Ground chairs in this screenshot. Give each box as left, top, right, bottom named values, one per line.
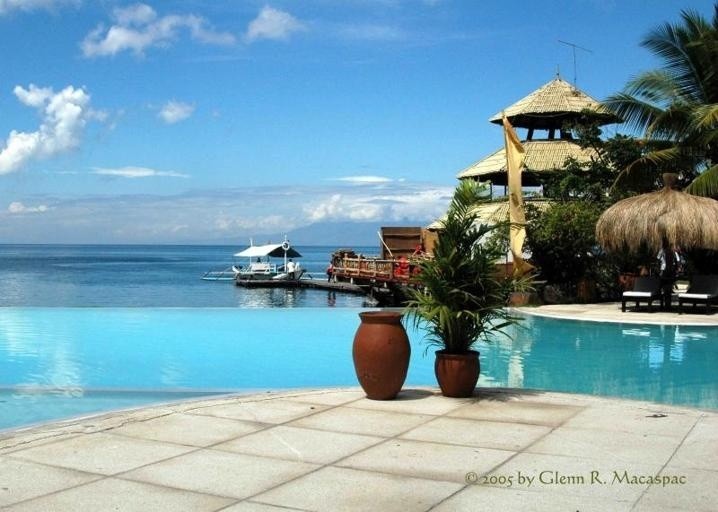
left=622, top=273, right=718, bottom=315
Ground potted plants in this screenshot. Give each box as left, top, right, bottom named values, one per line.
left=397, top=176, right=549, bottom=398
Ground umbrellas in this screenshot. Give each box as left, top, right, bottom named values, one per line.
left=596, top=184, right=718, bottom=312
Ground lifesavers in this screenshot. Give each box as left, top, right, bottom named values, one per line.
left=282, top=241, right=290, bottom=251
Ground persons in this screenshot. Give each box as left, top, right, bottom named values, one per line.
left=286, top=259, right=295, bottom=280
left=327, top=261, right=336, bottom=283
left=656, top=247, right=680, bottom=292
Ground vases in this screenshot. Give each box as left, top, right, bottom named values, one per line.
left=353, top=312, right=411, bottom=399
